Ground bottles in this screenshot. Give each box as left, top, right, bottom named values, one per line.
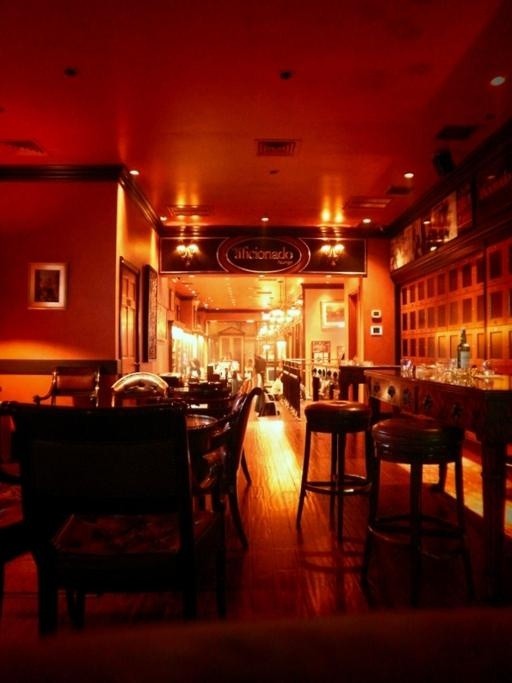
left=456, top=329, right=470, bottom=368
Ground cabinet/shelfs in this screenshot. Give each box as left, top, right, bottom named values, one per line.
left=280, top=358, right=305, bottom=415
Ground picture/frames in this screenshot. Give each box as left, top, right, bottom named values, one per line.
left=156, top=301, right=169, bottom=344
left=319, top=299, right=345, bottom=331
left=142, top=264, right=157, bottom=362
left=27, top=256, right=71, bottom=314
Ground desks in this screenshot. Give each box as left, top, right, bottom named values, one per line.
left=0, top=388, right=223, bottom=576
left=310, top=361, right=401, bottom=435
left=364, top=365, right=512, bottom=604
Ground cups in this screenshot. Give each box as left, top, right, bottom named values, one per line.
left=400, top=358, right=496, bottom=387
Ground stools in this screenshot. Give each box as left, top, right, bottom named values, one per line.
left=294, top=391, right=379, bottom=546
left=355, top=415, right=478, bottom=606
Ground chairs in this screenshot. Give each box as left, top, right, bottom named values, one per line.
left=11, top=388, right=217, bottom=637
left=32, top=364, right=99, bottom=406
left=191, top=370, right=268, bottom=558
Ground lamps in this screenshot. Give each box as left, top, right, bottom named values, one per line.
left=322, top=237, right=345, bottom=267
left=176, top=240, right=205, bottom=267
